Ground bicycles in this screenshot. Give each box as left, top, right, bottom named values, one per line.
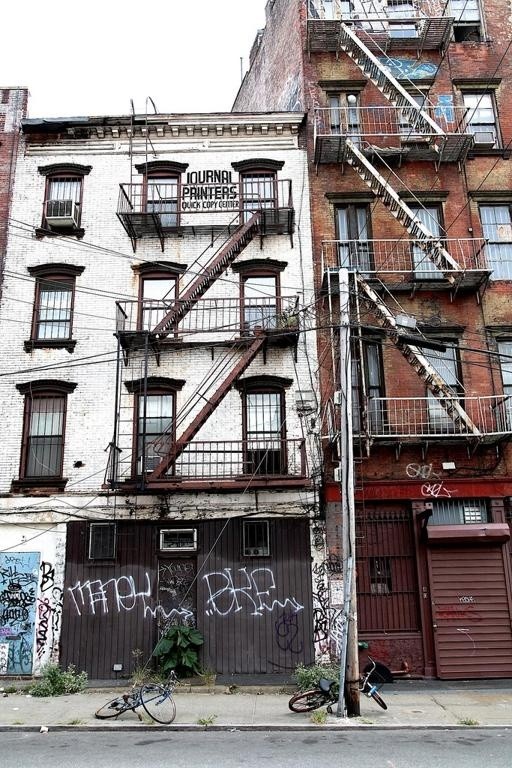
left=286, top=653, right=396, bottom=715
left=92, top=667, right=183, bottom=725
